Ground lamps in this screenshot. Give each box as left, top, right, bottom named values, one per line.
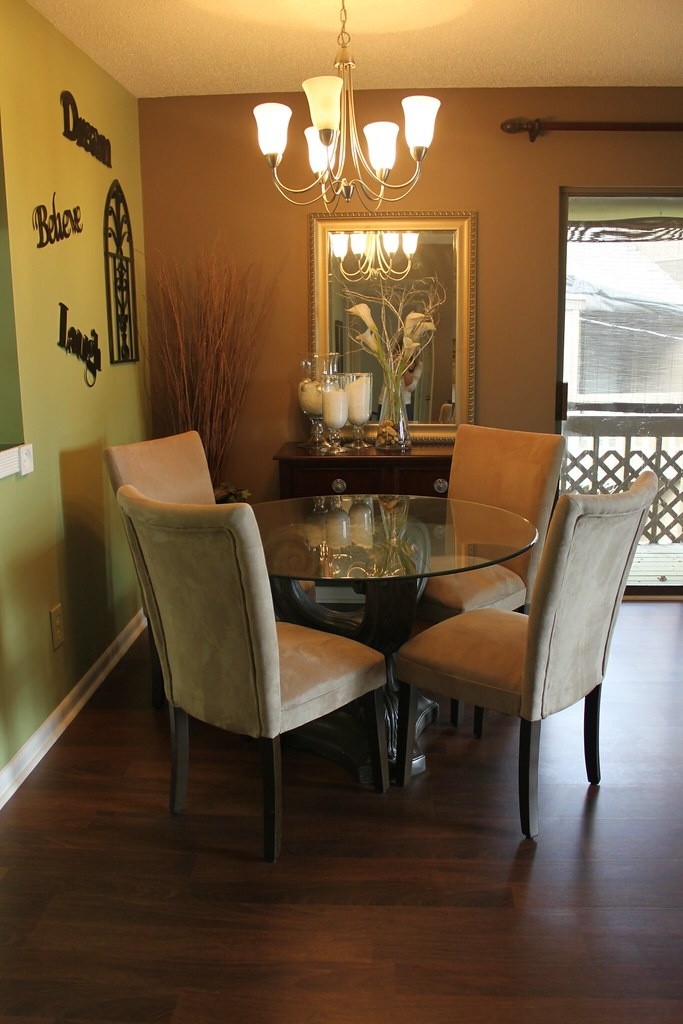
left=330, top=221, right=420, bottom=284
left=251, top=1, right=441, bottom=214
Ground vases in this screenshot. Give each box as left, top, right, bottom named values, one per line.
left=381, top=377, right=410, bottom=452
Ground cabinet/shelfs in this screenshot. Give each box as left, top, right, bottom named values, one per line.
left=272, top=441, right=452, bottom=499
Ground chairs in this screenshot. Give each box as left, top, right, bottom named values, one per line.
left=105, top=430, right=216, bottom=713
left=432, top=426, right=566, bottom=741
left=114, top=483, right=389, bottom=865
left=396, top=472, right=659, bottom=837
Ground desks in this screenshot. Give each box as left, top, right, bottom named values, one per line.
left=227, top=497, right=539, bottom=787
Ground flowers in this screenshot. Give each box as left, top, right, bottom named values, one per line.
left=346, top=289, right=442, bottom=373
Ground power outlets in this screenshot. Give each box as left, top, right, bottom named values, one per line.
left=51, top=603, right=66, bottom=650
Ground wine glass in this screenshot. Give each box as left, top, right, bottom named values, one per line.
left=306, top=495, right=374, bottom=552
left=321, top=374, right=349, bottom=454
left=294, top=351, right=340, bottom=449
left=343, top=371, right=373, bottom=450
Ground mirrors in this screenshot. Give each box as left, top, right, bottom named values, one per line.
left=310, top=214, right=478, bottom=447
